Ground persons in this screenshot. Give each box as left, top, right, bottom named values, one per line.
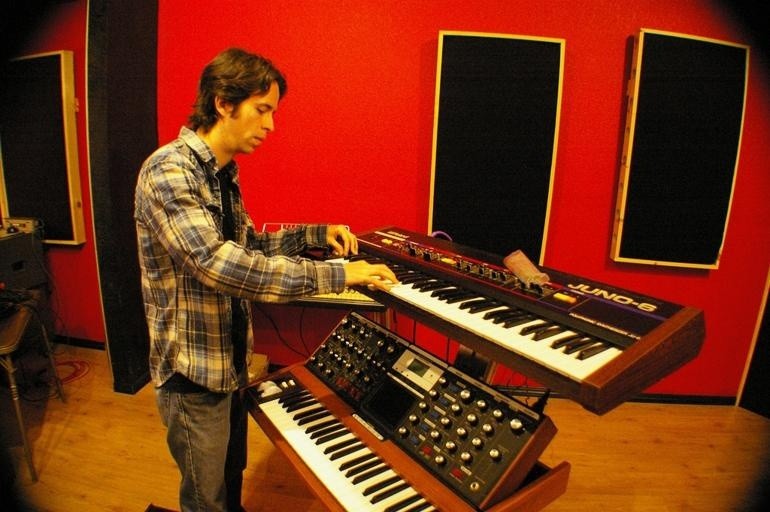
left=134, top=46, right=402, bottom=511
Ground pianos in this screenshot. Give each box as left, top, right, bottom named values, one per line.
left=237, top=227, right=706, bottom=512
